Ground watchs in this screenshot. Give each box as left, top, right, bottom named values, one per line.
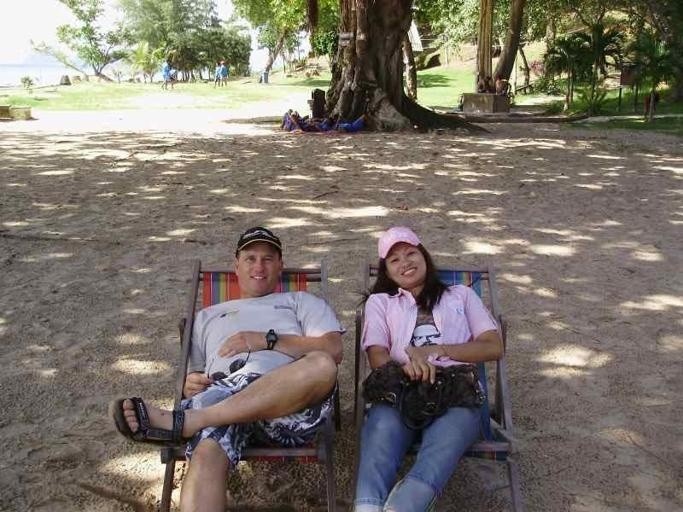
left=265, top=329, right=278, bottom=350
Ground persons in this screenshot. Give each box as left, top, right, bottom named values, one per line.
left=214, top=61, right=221, bottom=87
left=160, top=59, right=172, bottom=91
left=347, top=225, right=504, bottom=511
left=219, top=61, right=228, bottom=87
left=111, top=226, right=346, bottom=512
left=169, top=69, right=178, bottom=89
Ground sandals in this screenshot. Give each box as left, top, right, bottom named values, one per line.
left=112, top=396, right=193, bottom=446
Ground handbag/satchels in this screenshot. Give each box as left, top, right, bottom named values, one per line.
left=363, top=357, right=485, bottom=427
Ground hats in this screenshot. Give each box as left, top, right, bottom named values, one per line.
left=236, top=226, right=283, bottom=252
left=378, top=225, right=421, bottom=257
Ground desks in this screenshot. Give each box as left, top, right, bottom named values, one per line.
left=464, top=93, right=508, bottom=113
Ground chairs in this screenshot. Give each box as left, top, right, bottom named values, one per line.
left=159, top=259, right=341, bottom=511
left=354, top=262, right=522, bottom=512
